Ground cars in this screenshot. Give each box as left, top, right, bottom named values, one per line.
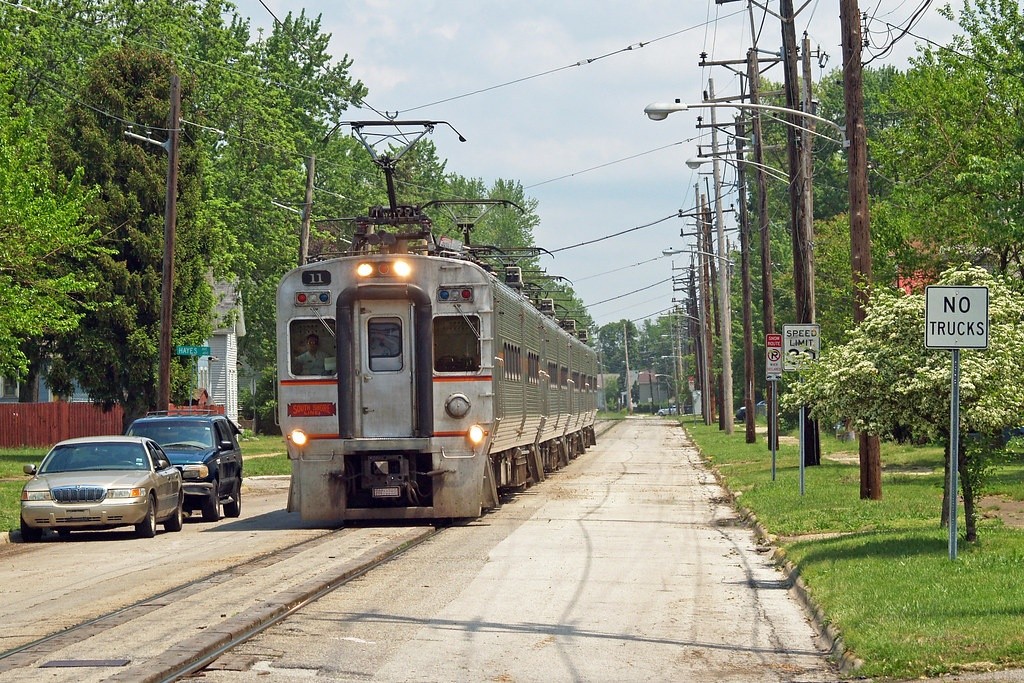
left=20, top=435, right=185, bottom=542
left=736, top=398, right=769, bottom=419
left=658, top=400, right=693, bottom=417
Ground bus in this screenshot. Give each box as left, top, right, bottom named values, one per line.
left=275, top=114, right=600, bottom=521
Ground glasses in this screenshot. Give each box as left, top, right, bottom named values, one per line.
left=307, top=341, right=319, bottom=346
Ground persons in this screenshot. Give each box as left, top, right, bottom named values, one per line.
left=297, top=334, right=333, bottom=375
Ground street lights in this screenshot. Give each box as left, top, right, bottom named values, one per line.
left=643, top=99, right=885, bottom=502
left=662, top=247, right=735, bottom=435
left=685, top=156, right=822, bottom=469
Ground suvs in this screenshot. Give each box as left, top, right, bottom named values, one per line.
left=125, top=408, right=244, bottom=521
